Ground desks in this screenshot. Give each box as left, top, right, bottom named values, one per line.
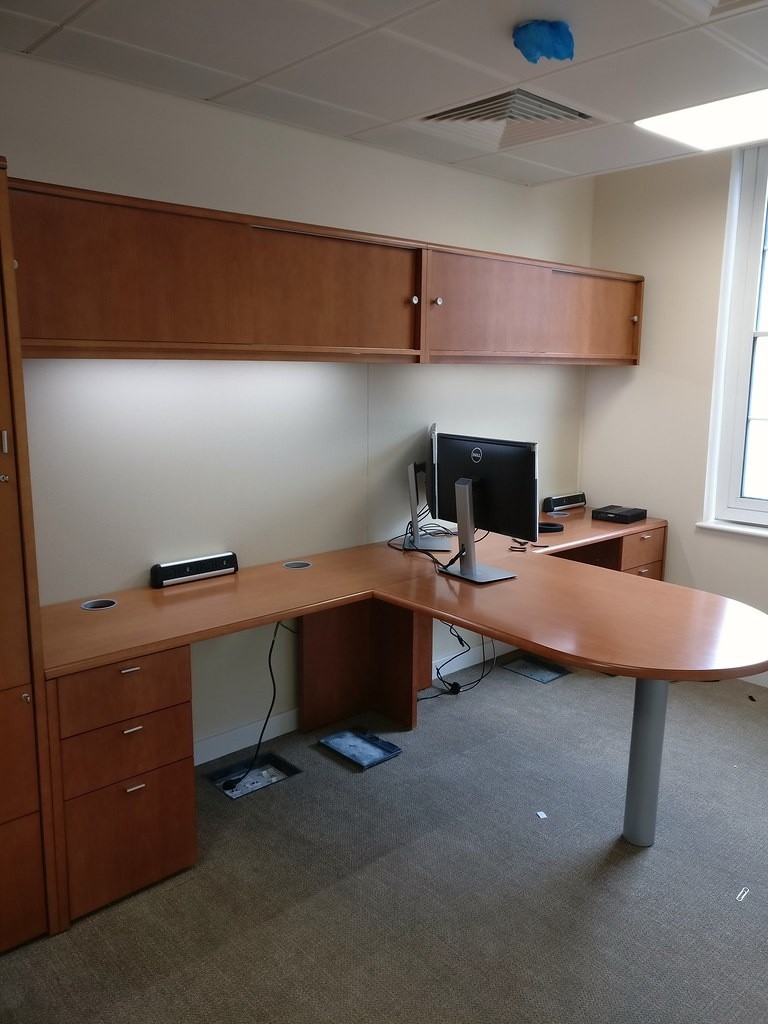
left=371, top=550, right=768, bottom=847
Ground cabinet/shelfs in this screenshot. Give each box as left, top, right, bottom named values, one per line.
left=0, top=155, right=670, bottom=959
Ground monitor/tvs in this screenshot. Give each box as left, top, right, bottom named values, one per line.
left=403, top=423, right=539, bottom=584
